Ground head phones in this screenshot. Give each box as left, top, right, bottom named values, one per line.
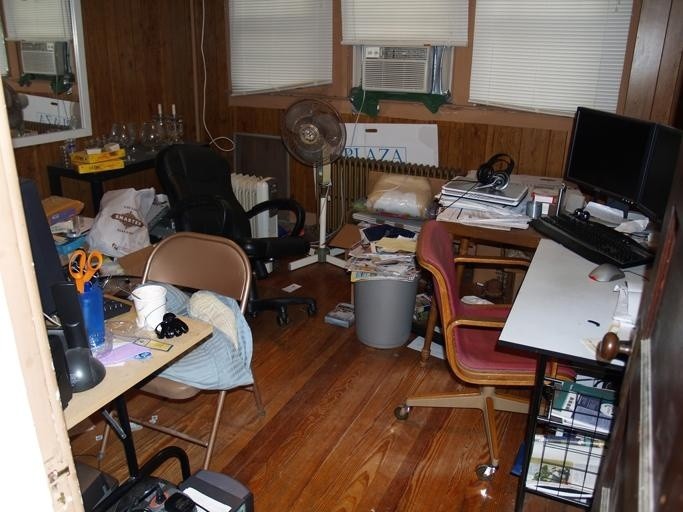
left=476, top=153, right=515, bottom=191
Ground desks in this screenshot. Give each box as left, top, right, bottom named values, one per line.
left=46, top=133, right=172, bottom=225
left=414, top=206, right=549, bottom=370
left=35, top=285, right=214, bottom=512
left=495, top=226, right=650, bottom=510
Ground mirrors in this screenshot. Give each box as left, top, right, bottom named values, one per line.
left=0, top=0, right=93, bottom=150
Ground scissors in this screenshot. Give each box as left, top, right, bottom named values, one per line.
left=68, top=250, right=103, bottom=293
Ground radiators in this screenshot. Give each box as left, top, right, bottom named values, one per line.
left=319, top=151, right=461, bottom=235
left=23, top=120, right=71, bottom=138
left=229, top=171, right=275, bottom=275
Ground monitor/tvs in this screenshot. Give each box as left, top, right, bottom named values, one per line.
left=563, top=107, right=683, bottom=226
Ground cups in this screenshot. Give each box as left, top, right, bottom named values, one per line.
left=72, top=214, right=84, bottom=228
left=80, top=286, right=105, bottom=348
left=84, top=119, right=185, bottom=155
left=525, top=200, right=541, bottom=220
left=65, top=221, right=80, bottom=239
left=131, top=285, right=167, bottom=330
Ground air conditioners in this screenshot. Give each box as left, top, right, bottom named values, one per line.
left=20, top=39, right=65, bottom=77
left=358, top=41, right=435, bottom=96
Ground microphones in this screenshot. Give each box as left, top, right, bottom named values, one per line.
left=475, top=178, right=498, bottom=190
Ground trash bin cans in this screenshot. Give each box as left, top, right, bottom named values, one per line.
left=354, top=274, right=422, bottom=349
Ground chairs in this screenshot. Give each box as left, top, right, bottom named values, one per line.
left=94, top=232, right=253, bottom=477
left=154, top=142, right=318, bottom=327
left=392, top=216, right=578, bottom=479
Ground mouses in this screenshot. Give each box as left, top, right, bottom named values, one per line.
left=589, top=263, right=625, bottom=282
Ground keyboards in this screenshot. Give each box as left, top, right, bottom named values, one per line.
left=532, top=212, right=655, bottom=269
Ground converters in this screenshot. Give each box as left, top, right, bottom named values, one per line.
left=165, top=493, right=195, bottom=512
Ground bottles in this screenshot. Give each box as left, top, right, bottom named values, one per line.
left=58, top=137, right=75, bottom=167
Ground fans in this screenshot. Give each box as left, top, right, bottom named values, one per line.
left=2, top=82, right=25, bottom=139
left=279, top=98, right=355, bottom=274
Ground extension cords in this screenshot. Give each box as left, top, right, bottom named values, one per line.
left=148, top=485, right=178, bottom=512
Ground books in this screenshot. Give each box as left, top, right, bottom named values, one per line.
left=435, top=175, right=532, bottom=231
left=525, top=384, right=614, bottom=507
left=324, top=302, right=354, bottom=328
left=345, top=245, right=419, bottom=281
left=408, top=293, right=446, bottom=359
